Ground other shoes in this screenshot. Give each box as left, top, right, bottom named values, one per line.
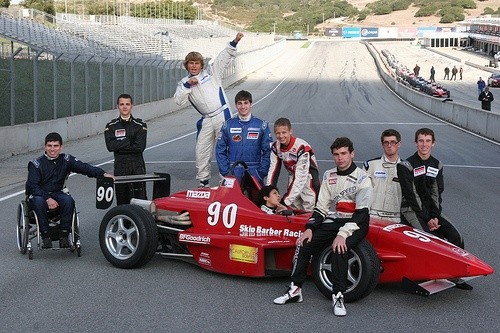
left=40, top=225, right=53, bottom=248
left=198, top=180, right=210, bottom=189
left=59, top=227, right=71, bottom=248
left=454, top=282, right=473, bottom=290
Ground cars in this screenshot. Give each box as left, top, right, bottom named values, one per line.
left=489, top=50, right=496, bottom=57
left=494, top=51, right=500, bottom=61
left=487, top=72, right=500, bottom=88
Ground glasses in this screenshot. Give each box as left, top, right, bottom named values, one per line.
left=382, top=140, right=400, bottom=146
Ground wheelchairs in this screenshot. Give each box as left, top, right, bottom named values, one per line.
left=16, top=180, right=82, bottom=261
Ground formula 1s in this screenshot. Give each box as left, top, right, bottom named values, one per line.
left=94, top=157, right=495, bottom=303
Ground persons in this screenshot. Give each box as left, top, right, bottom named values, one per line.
left=216, top=90, right=273, bottom=207
left=25, top=132, right=116, bottom=249
left=172, top=31, right=244, bottom=189
left=258, top=186, right=287, bottom=215
left=397, top=128, right=474, bottom=290
left=459, top=68, right=463, bottom=80
left=477, top=77, right=495, bottom=112
left=402, top=68, right=441, bottom=89
left=450, top=65, right=459, bottom=80
left=429, top=66, right=436, bottom=84
left=361, top=129, right=403, bottom=225
left=443, top=66, right=450, bottom=80
left=272, top=136, right=373, bottom=316
left=265, top=117, right=319, bottom=211
left=414, top=64, right=421, bottom=76
left=104, top=93, right=148, bottom=205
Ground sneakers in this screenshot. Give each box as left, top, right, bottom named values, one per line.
left=331, top=291, right=346, bottom=315
left=273, top=282, right=303, bottom=304
